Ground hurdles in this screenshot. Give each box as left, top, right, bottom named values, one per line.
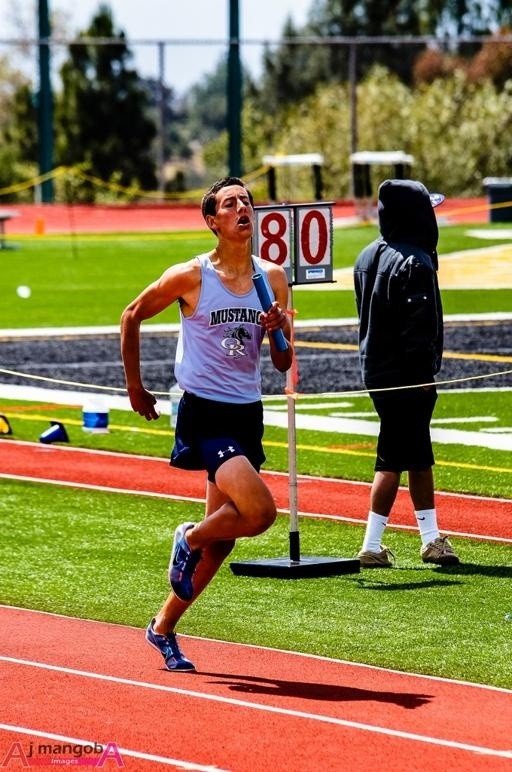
left=349, top=150, right=415, bottom=212
left=262, top=154, right=324, bottom=206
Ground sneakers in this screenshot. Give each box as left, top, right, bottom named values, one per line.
left=168, top=521, right=204, bottom=605
left=145, top=616, right=196, bottom=673
left=356, top=544, right=393, bottom=567
left=420, top=537, right=459, bottom=564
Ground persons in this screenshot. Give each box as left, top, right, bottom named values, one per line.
left=352, top=180, right=461, bottom=570
left=119, top=178, right=296, bottom=671
